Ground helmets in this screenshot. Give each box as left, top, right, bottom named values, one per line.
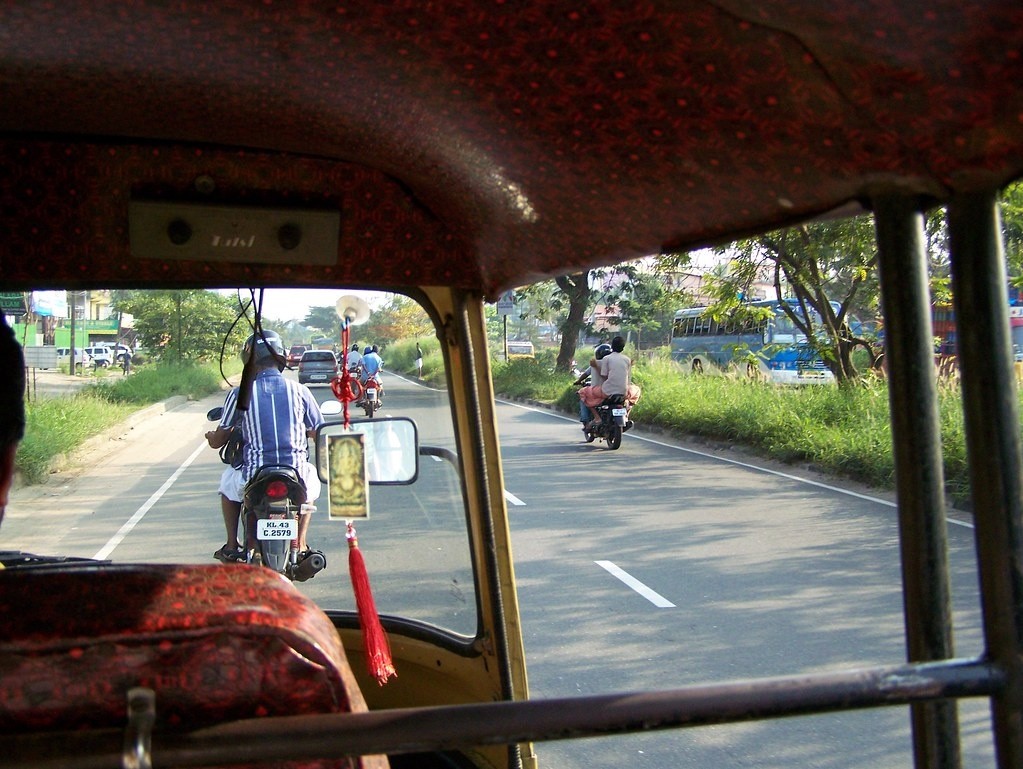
left=351, top=344, right=359, bottom=351
left=364, top=347, right=371, bottom=354
left=594, top=344, right=613, bottom=359
left=241, top=331, right=286, bottom=373
left=372, top=345, right=378, bottom=353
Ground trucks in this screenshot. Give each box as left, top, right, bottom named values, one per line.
left=507, top=341, right=535, bottom=366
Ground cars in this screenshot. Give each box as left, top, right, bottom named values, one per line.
left=298, top=350, right=341, bottom=387
left=288, top=334, right=339, bottom=367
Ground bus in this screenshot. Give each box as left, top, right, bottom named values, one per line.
left=930, top=286, right=1022, bottom=389
left=668, top=297, right=852, bottom=385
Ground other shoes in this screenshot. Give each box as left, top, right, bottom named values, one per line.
left=289, top=545, right=312, bottom=562
left=589, top=420, right=602, bottom=426
left=354, top=399, right=362, bottom=403
left=377, top=399, right=381, bottom=404
left=582, top=427, right=592, bottom=432
left=214, top=544, right=237, bottom=564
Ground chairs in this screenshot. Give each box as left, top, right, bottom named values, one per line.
left=0, top=564, right=391, bottom=769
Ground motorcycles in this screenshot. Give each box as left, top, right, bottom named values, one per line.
left=348, top=366, right=383, bottom=419
left=573, top=377, right=634, bottom=450
left=207, top=399, right=343, bottom=583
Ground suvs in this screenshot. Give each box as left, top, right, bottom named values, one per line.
left=39, top=347, right=91, bottom=370
left=101, top=343, right=133, bottom=362
left=85, top=346, right=113, bottom=368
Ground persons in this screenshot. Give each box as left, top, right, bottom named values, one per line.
left=0, top=305, right=26, bottom=532
left=574, top=343, right=612, bottom=432
left=123, top=349, right=131, bottom=377
left=578, top=336, right=641, bottom=426
left=415, top=343, right=423, bottom=380
left=332, top=343, right=384, bottom=411
left=205, top=329, right=325, bottom=560
